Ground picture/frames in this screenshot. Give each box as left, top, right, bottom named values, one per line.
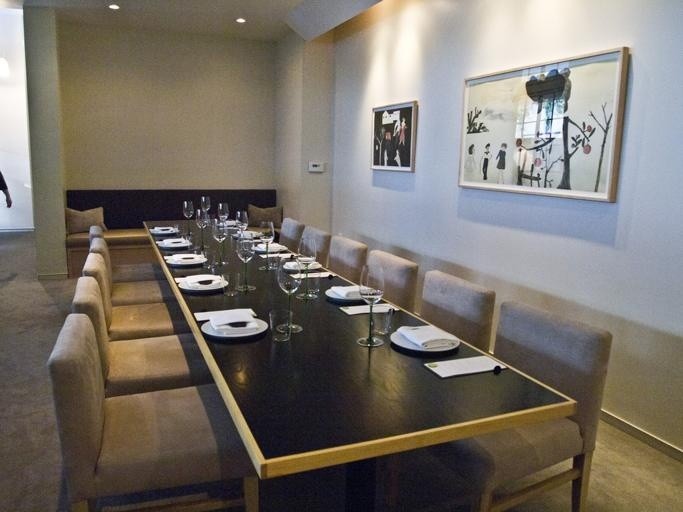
left=458, top=46, right=629, bottom=202
left=369, top=100, right=417, bottom=172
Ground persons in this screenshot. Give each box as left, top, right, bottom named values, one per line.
left=0, top=172, right=12, bottom=208
left=398, top=118, right=406, bottom=146
left=464, top=138, right=526, bottom=184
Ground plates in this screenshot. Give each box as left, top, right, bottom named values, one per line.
left=325, top=289, right=342, bottom=301
left=178, top=280, right=229, bottom=291
left=157, top=242, right=192, bottom=248
left=200, top=318, right=268, bottom=339
left=150, top=229, right=180, bottom=235
left=166, top=258, right=207, bottom=265
left=390, top=331, right=461, bottom=353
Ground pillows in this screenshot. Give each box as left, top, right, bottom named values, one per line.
left=66, top=207, right=108, bottom=234
left=247, top=203, right=283, bottom=227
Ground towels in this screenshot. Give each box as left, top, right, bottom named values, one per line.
left=258, top=243, right=279, bottom=251
left=226, top=221, right=236, bottom=225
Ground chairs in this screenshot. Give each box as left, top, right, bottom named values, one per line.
left=87, top=226, right=167, bottom=283
left=48, top=312, right=247, bottom=511
left=456, top=300, right=611, bottom=510
left=298, top=225, right=333, bottom=270
left=281, top=218, right=305, bottom=254
left=325, top=234, right=371, bottom=286
left=419, top=270, right=497, bottom=354
left=89, top=237, right=179, bottom=306
left=81, top=253, right=195, bottom=342
left=362, top=250, right=419, bottom=315
left=70, top=274, right=214, bottom=398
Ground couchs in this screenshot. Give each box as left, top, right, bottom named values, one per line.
left=66, top=189, right=279, bottom=279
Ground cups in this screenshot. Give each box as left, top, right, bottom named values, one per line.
left=202, top=249, right=216, bottom=269
left=269, top=310, right=293, bottom=342
left=222, top=272, right=239, bottom=296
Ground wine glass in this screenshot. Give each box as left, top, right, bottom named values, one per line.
left=236, top=232, right=257, bottom=291
left=277, top=237, right=320, bottom=335
left=356, top=263, right=385, bottom=348
left=183, top=196, right=249, bottom=266
left=259, top=222, right=278, bottom=271
